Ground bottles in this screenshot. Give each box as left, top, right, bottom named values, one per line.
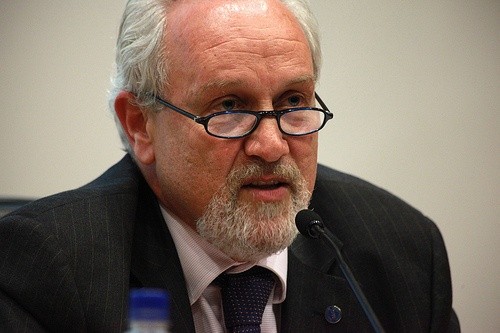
left=128, top=290, right=170, bottom=333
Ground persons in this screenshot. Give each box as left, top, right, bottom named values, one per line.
left=0, top=0, right=462, bottom=333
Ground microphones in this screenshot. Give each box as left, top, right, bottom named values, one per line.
left=296, top=209, right=385, bottom=333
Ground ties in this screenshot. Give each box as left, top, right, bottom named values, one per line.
left=211, top=265, right=276, bottom=333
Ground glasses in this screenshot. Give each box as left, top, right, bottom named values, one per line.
left=151, top=92, right=333, bottom=139
